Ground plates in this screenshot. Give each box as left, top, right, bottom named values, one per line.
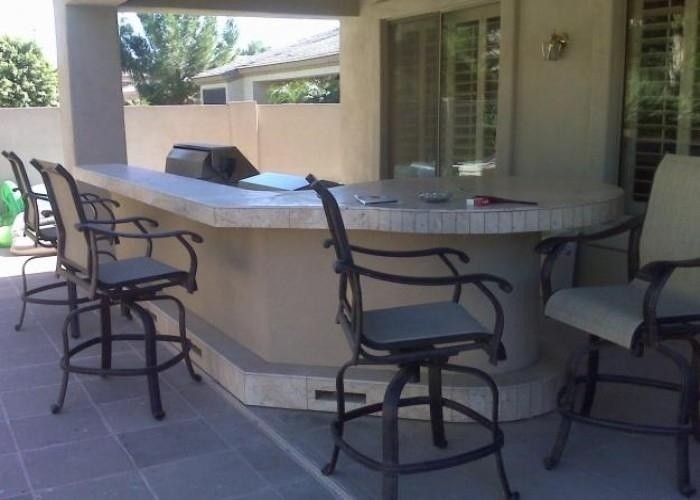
left=415, top=192, right=452, bottom=202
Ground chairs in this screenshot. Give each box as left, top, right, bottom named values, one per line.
left=3, top=152, right=132, bottom=338
left=304, top=172, right=522, bottom=499
left=29, top=158, right=202, bottom=419
left=532, top=149, right=699, bottom=497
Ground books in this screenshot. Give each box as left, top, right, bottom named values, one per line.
left=353, top=192, right=398, bottom=205
